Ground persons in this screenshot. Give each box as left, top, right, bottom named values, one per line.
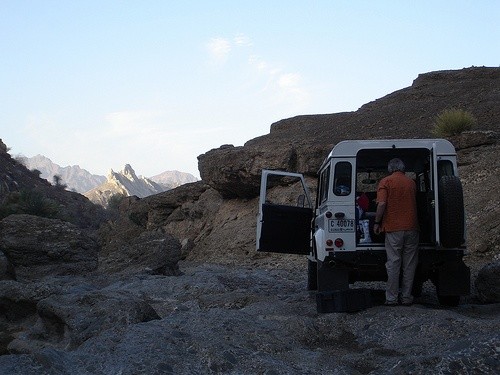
left=373, top=158, right=419, bottom=306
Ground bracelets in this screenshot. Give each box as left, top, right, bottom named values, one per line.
left=375, top=222, right=380, bottom=224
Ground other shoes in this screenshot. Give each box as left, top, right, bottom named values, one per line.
left=401, top=299, right=416, bottom=305
left=384, top=301, right=399, bottom=306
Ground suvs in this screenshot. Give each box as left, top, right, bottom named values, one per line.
left=255, top=139, right=466, bottom=306
